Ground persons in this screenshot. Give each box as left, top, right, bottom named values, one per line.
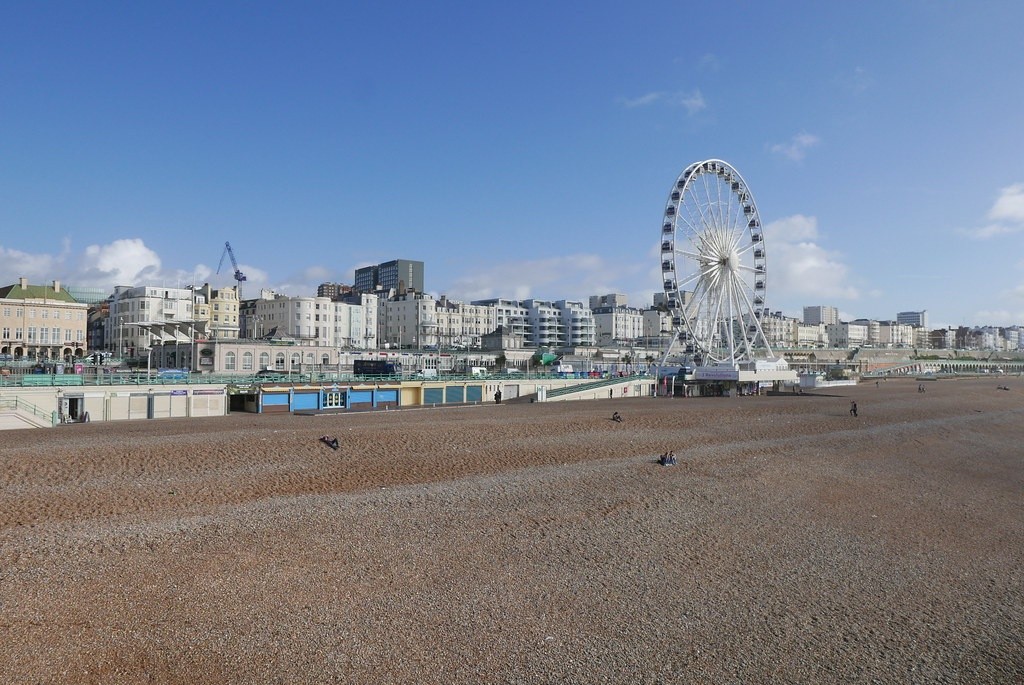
left=661, top=451, right=678, bottom=467
left=918, top=384, right=926, bottom=394
left=885, top=378, right=888, bottom=383
left=609, top=388, right=613, bottom=400
left=849, top=400, right=858, bottom=417
left=996, top=384, right=1011, bottom=391
left=876, top=381, right=879, bottom=388
left=613, top=412, right=622, bottom=422
left=318, top=434, right=339, bottom=450
left=495, top=390, right=502, bottom=404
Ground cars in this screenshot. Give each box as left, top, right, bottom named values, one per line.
left=2, top=339, right=692, bottom=386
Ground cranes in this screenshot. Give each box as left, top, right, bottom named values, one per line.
left=218, top=241, right=247, bottom=298
